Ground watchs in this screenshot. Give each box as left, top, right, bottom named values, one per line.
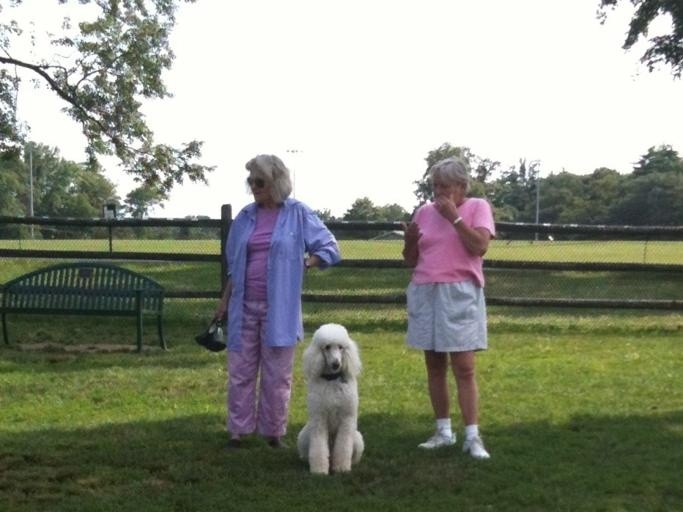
left=303, top=258, right=309, bottom=269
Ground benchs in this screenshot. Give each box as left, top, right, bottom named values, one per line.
left=0, top=263, right=167, bottom=352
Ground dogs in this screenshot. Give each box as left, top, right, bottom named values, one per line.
left=296, top=323, right=364, bottom=475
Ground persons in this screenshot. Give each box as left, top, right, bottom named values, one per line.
left=194, top=154, right=340, bottom=446
left=399, top=155, right=494, bottom=462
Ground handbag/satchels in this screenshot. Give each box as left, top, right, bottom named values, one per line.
left=194, top=318, right=227, bottom=352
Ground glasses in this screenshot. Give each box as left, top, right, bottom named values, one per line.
left=247, top=177, right=266, bottom=188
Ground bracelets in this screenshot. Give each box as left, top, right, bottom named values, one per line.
left=452, top=215, right=462, bottom=226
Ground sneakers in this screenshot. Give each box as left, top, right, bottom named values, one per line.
left=224, top=438, right=246, bottom=452
left=267, top=438, right=289, bottom=451
left=416, top=433, right=457, bottom=450
left=461, top=437, right=492, bottom=462
